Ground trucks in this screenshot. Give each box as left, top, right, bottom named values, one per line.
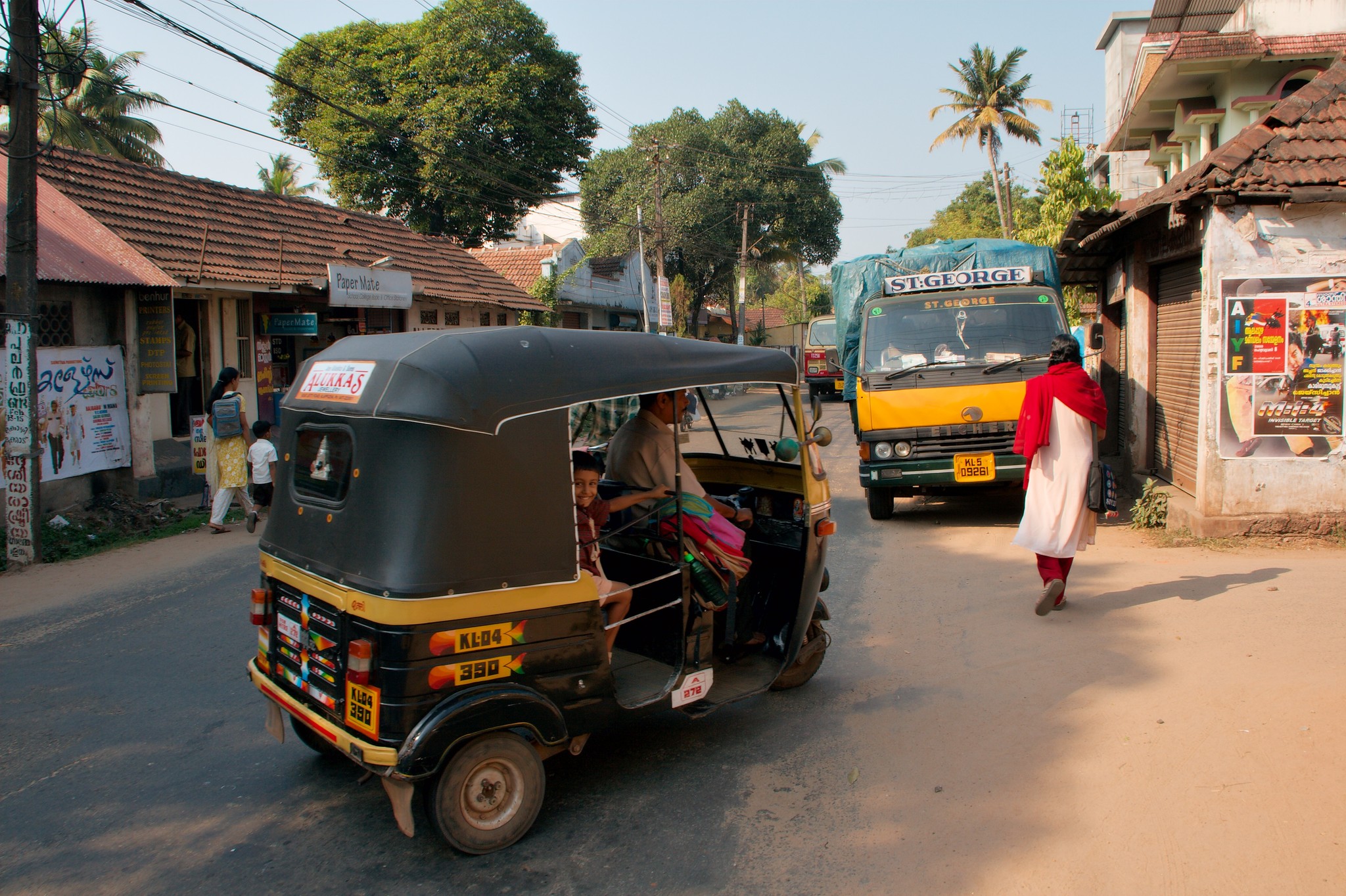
left=830, top=238, right=1106, bottom=519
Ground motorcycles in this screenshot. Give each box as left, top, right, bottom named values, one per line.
left=243, top=324, right=833, bottom=853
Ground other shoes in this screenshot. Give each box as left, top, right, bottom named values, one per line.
left=1052, top=595, right=1067, bottom=610
left=58, top=461, right=62, bottom=469
left=78, top=460, right=81, bottom=467
left=54, top=468, right=58, bottom=474
left=247, top=512, right=257, bottom=533
left=1035, top=579, right=1064, bottom=616
left=72, top=455, right=76, bottom=465
left=687, top=415, right=693, bottom=421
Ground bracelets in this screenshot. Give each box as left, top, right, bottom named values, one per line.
left=1329, top=279, right=1334, bottom=288
left=730, top=507, right=738, bottom=520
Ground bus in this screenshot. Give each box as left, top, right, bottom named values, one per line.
left=805, top=312, right=848, bottom=402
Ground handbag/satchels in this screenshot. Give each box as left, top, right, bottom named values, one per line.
left=1087, top=461, right=1117, bottom=513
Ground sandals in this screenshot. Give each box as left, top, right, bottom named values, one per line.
left=718, top=630, right=768, bottom=652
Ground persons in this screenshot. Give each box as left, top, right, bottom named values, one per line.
left=1021, top=335, right=1107, bottom=617
left=572, top=450, right=674, bottom=684
left=1226, top=278, right=1346, bottom=457
left=205, top=367, right=278, bottom=535
left=40, top=400, right=86, bottom=475
left=605, top=389, right=768, bottom=650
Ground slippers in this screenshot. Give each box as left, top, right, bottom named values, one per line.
left=210, top=526, right=231, bottom=534
left=256, top=516, right=261, bottom=521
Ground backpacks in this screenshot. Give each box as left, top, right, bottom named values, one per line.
left=212, top=392, right=244, bottom=438
left=654, top=493, right=752, bottom=609
